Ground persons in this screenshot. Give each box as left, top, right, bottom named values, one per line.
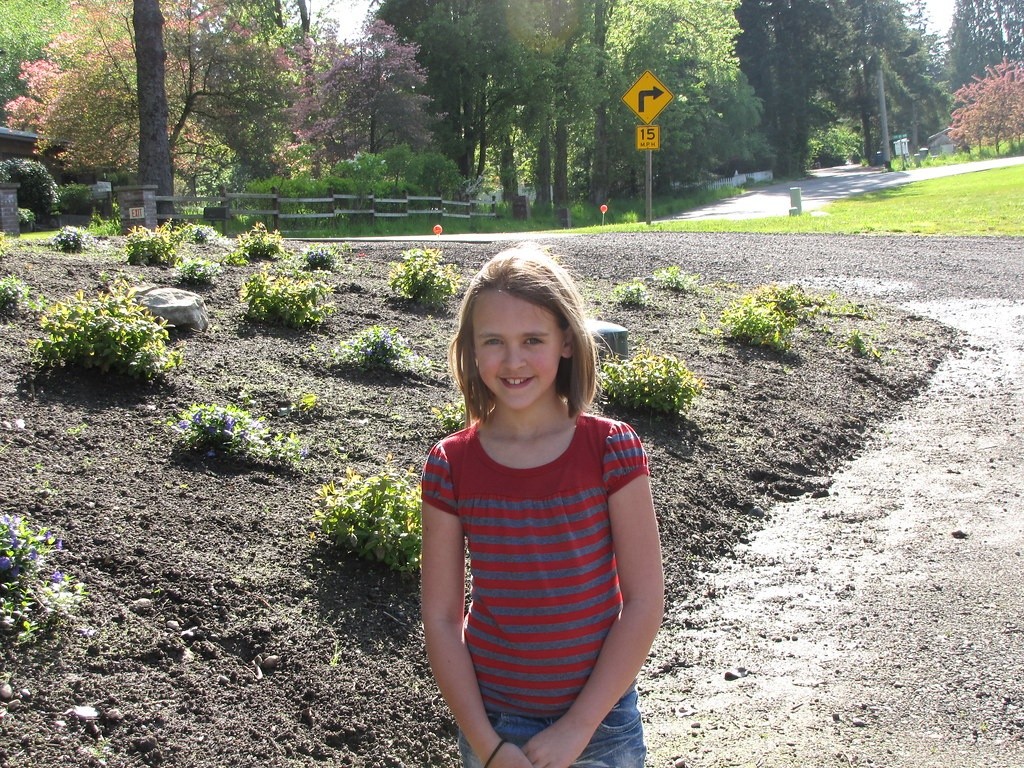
left=421, top=247, right=665, bottom=768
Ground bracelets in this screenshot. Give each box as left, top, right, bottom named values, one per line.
left=483, top=739, right=507, bottom=768
